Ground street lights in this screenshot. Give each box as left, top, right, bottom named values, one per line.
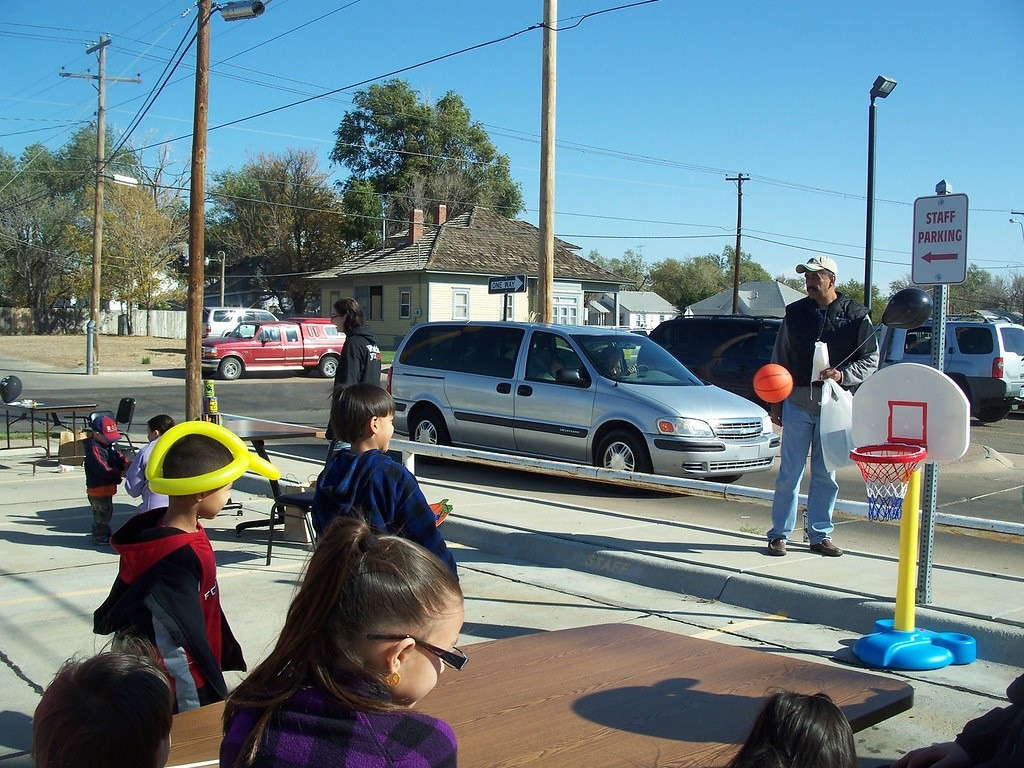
left=864, top=75, right=899, bottom=319
left=204, top=250, right=225, bottom=308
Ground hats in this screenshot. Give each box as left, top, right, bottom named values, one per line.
left=795, top=255, right=838, bottom=276
left=92, top=416, right=122, bottom=443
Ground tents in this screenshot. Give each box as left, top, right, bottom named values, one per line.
left=686, top=280, right=808, bottom=322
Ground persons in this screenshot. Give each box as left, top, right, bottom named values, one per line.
left=726, top=692, right=858, bottom=768
left=218, top=517, right=465, bottom=768
left=125, top=414, right=175, bottom=513
left=326, top=297, right=381, bottom=464
left=83, top=415, right=128, bottom=545
left=311, top=384, right=458, bottom=581
left=32, top=650, right=174, bottom=768
left=876, top=673, right=1024, bottom=768
left=93, top=434, right=247, bottom=715
left=767, top=255, right=880, bottom=554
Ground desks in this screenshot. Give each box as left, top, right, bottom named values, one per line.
left=0, top=399, right=100, bottom=476
left=212, top=418, right=325, bottom=541
left=163, top=622, right=916, bottom=768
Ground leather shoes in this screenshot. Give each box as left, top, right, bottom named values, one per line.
left=768, top=539, right=787, bottom=557
left=811, top=538, right=843, bottom=556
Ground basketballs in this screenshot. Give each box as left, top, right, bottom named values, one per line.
left=753, top=364, right=794, bottom=403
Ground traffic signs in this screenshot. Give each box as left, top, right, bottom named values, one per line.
left=910, top=194, right=970, bottom=287
left=487, top=274, right=529, bottom=295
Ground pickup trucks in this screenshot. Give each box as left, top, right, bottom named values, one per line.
left=200, top=316, right=346, bottom=380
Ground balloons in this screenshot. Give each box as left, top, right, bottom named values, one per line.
left=0, top=375, right=22, bottom=403
left=145, top=420, right=281, bottom=495
left=882, top=287, right=933, bottom=330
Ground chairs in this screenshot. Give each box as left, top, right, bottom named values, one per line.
left=265, top=489, right=317, bottom=566
left=81, top=395, right=138, bottom=462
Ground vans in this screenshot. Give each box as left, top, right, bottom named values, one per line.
left=201, top=307, right=281, bottom=342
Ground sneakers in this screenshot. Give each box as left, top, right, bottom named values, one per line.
left=93, top=535, right=109, bottom=544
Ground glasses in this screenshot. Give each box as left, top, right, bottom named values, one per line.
left=366, top=634, right=470, bottom=672
left=331, top=314, right=343, bottom=318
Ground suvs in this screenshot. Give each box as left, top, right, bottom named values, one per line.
left=873, top=310, right=1024, bottom=425
left=638, top=313, right=793, bottom=428
left=384, top=320, right=779, bottom=495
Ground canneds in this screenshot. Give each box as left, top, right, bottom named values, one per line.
left=202, top=379, right=223, bottom=426
